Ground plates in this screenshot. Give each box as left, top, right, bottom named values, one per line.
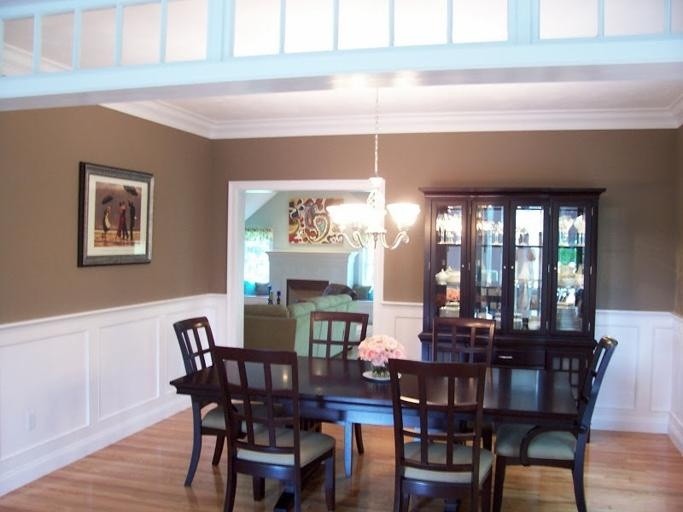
left=513, top=318, right=523, bottom=330
left=362, top=370, right=402, bottom=381
left=557, top=303, right=578, bottom=331
left=494, top=317, right=501, bottom=330
left=439, top=302, right=460, bottom=318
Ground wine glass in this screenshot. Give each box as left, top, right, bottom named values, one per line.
left=435, top=213, right=585, bottom=245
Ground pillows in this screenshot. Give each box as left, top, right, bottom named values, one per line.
left=352, top=282, right=372, bottom=299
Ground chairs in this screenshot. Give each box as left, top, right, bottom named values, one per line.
left=171, top=316, right=283, bottom=489
left=307, top=309, right=369, bottom=478
left=492, top=335, right=620, bottom=512
left=413, top=314, right=497, bottom=450
left=210, top=343, right=336, bottom=512
left=386, top=356, right=493, bottom=512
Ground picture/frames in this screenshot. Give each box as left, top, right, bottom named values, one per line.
left=75, top=159, right=155, bottom=267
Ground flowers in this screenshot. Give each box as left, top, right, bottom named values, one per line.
left=358, top=334, right=406, bottom=377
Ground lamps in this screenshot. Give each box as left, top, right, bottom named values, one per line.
left=327, top=91, right=420, bottom=249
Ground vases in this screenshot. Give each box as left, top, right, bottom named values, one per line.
left=371, top=365, right=386, bottom=377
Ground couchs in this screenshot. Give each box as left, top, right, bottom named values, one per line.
left=244, top=293, right=357, bottom=358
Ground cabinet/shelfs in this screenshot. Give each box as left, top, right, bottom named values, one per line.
left=431, top=194, right=509, bottom=332
left=508, top=199, right=593, bottom=333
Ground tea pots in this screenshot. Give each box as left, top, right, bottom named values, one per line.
left=435, top=268, right=450, bottom=285
